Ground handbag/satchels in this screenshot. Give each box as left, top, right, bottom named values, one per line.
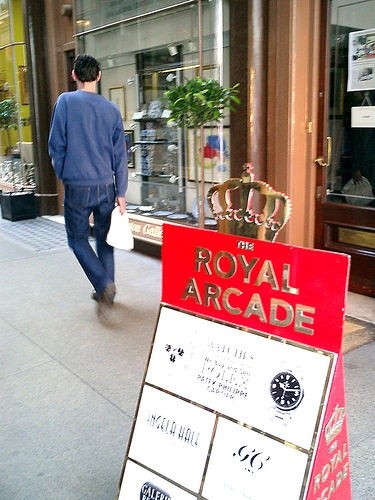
left=105, top=204, right=135, bottom=252
left=341, top=176, right=374, bottom=206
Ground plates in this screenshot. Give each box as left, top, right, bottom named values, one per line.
left=203, top=219, right=218, bottom=225
left=166, top=214, right=188, bottom=219
left=151, top=210, right=173, bottom=216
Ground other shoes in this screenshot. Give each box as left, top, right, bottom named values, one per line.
left=90, top=281, right=117, bottom=328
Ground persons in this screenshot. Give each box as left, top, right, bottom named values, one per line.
left=339, top=80, right=375, bottom=202
left=48, top=55, right=128, bottom=306
